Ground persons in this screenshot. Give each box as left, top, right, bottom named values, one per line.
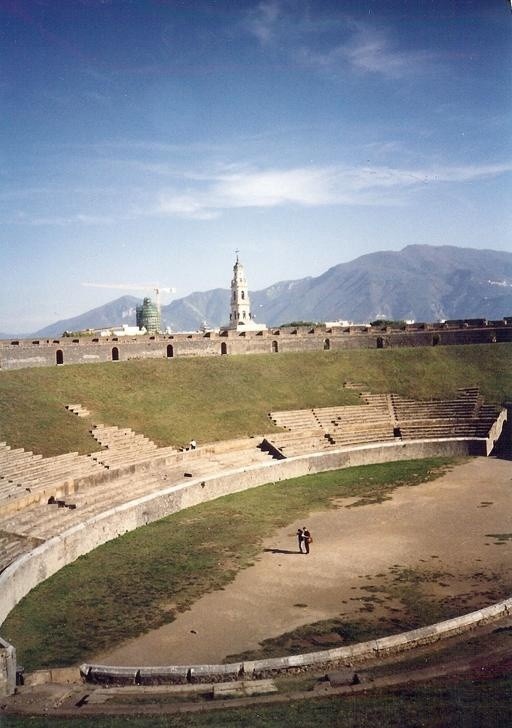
left=303, top=526, right=310, bottom=554
left=297, top=529, right=303, bottom=554
left=188, top=439, right=196, bottom=450
